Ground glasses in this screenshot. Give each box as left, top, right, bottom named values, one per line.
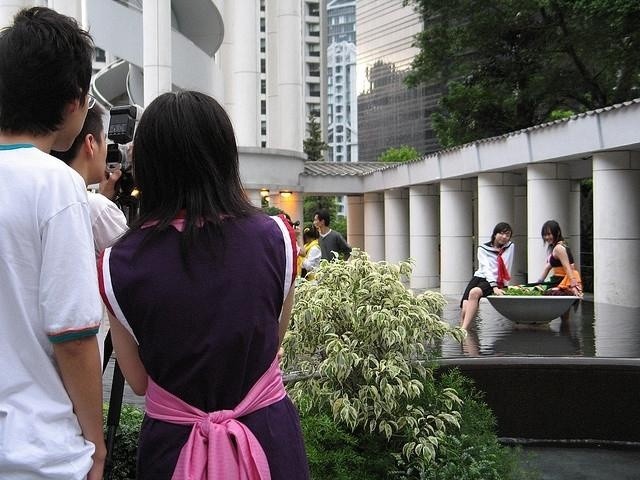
left=87, top=93, right=96, bottom=109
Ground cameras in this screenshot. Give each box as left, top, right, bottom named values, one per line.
left=292, top=220, right=300, bottom=229
left=105, top=105, right=137, bottom=173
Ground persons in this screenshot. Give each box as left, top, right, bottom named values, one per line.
left=460, top=222, right=515, bottom=328
left=301, top=210, right=350, bottom=282
left=1, top=5, right=311, bottom=480
left=535, top=220, right=584, bottom=332
